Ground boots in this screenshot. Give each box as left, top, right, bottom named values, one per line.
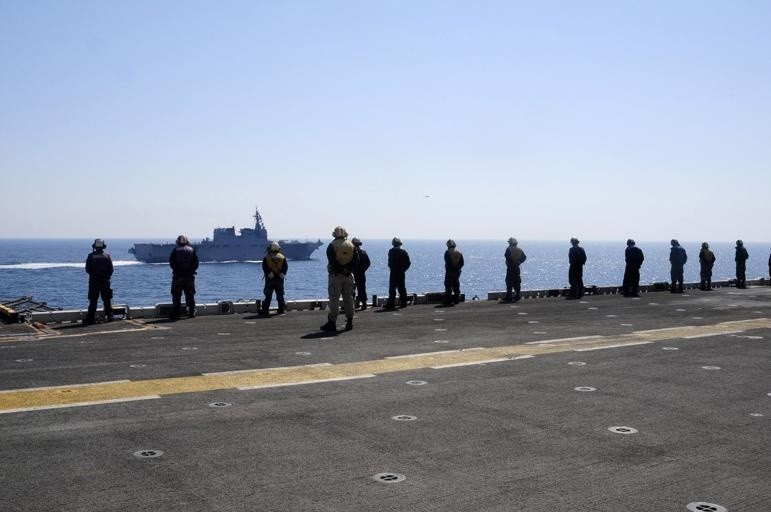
left=321, top=321, right=336, bottom=331
left=346, top=320, right=352, bottom=330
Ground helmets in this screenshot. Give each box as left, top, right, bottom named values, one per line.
left=176, top=236, right=187, bottom=245
left=447, top=240, right=456, bottom=248
left=392, top=238, right=401, bottom=246
left=271, top=242, right=280, bottom=253
left=508, top=238, right=517, bottom=245
left=333, top=227, right=347, bottom=238
left=352, top=237, right=362, bottom=245
left=92, top=239, right=105, bottom=249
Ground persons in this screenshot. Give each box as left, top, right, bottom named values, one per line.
left=169, top=233, right=199, bottom=318
left=85, top=238, right=115, bottom=321
left=341, top=237, right=372, bottom=311
left=381, top=236, right=411, bottom=309
left=767, top=251, right=771, bottom=287
left=622, top=239, right=644, bottom=294
left=733, top=239, right=750, bottom=290
left=261, top=241, right=289, bottom=315
left=698, top=242, right=718, bottom=292
left=564, top=237, right=588, bottom=299
left=320, top=224, right=360, bottom=332
left=440, top=238, right=465, bottom=304
left=668, top=238, right=688, bottom=294
left=501, top=236, right=527, bottom=301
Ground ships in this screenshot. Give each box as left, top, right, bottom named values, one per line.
left=127, top=206, right=325, bottom=264
